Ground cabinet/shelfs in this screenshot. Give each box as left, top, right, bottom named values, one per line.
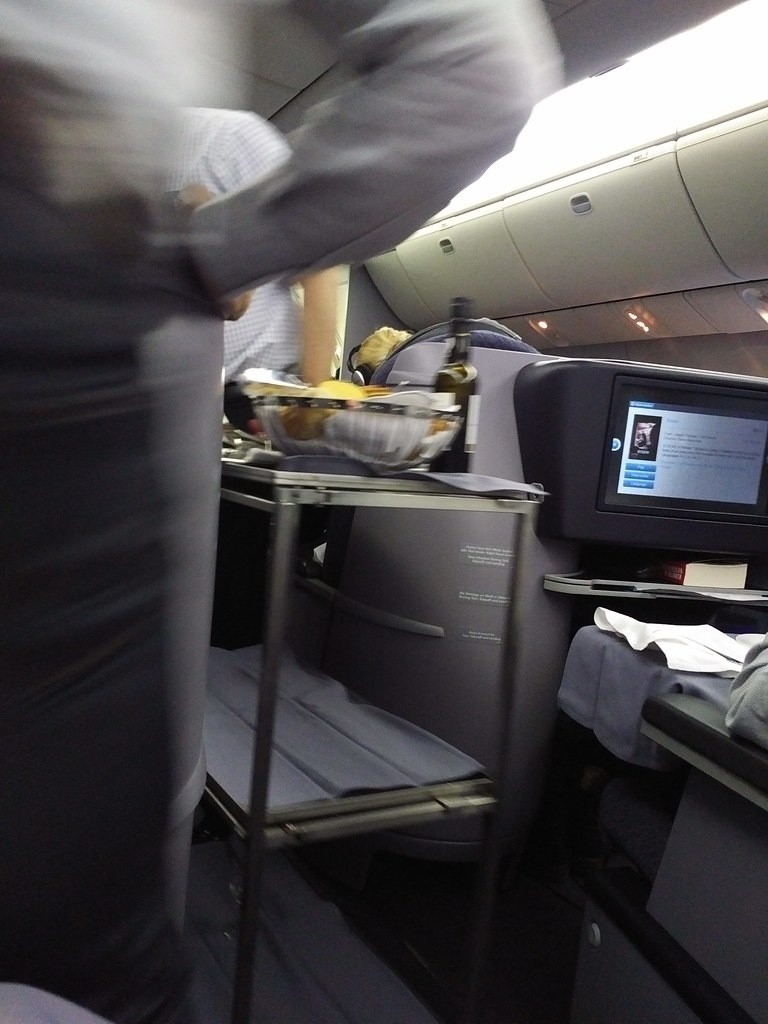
left=189, top=451, right=550, bottom=1023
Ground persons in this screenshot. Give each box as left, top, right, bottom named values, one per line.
left=1, top=0, right=562, bottom=1016
left=181, top=100, right=351, bottom=392
left=352, top=324, right=414, bottom=375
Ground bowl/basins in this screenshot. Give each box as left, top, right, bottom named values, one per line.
left=249, top=386, right=465, bottom=473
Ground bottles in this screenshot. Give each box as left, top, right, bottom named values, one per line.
left=425, top=299, right=480, bottom=476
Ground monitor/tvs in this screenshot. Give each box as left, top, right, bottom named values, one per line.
left=594, top=373, right=768, bottom=527
